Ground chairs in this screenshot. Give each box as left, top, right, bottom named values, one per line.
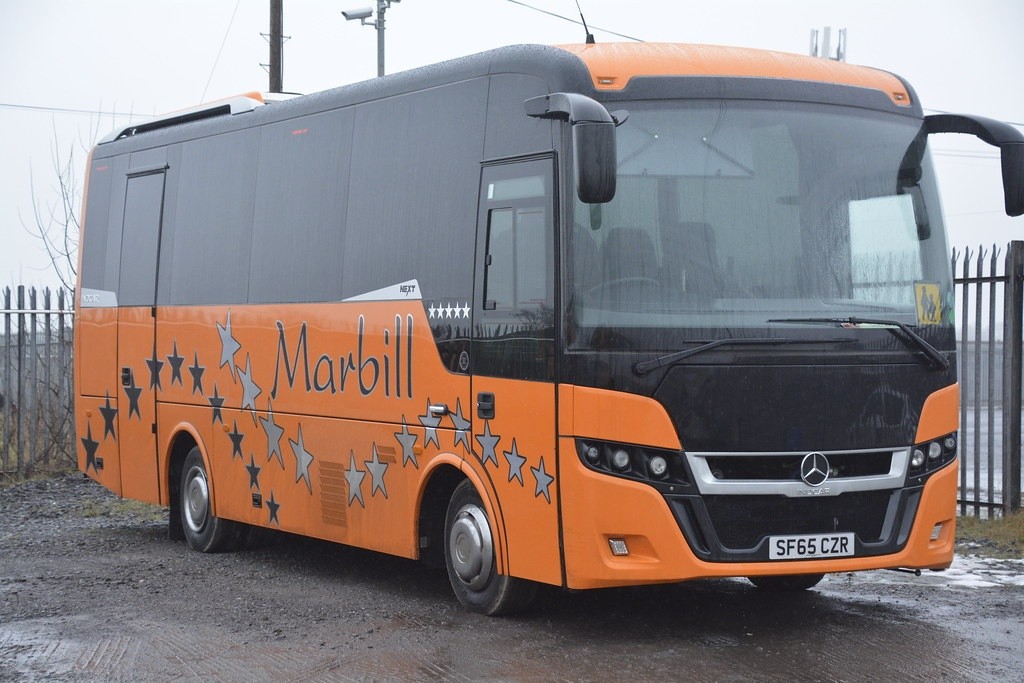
left=493, top=221, right=722, bottom=314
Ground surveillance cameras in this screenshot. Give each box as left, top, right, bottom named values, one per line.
left=341, top=7, right=373, bottom=21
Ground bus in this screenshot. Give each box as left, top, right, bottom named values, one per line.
left=71, top=39, right=1022, bottom=615
